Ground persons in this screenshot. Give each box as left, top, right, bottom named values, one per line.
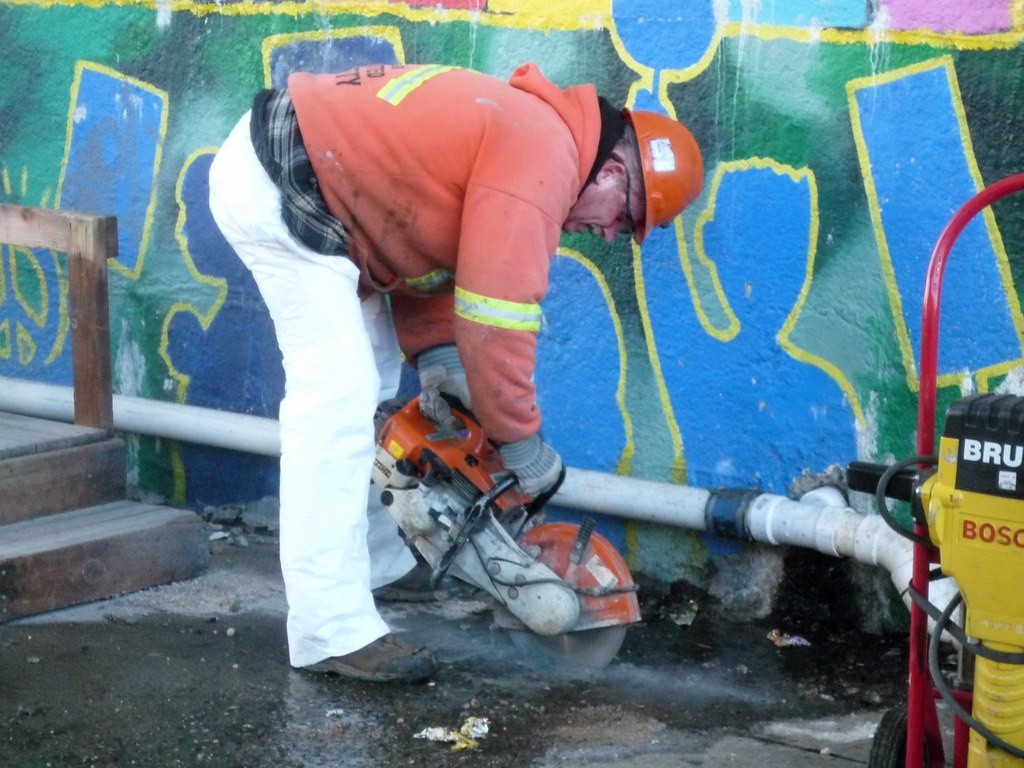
left=206, top=62, right=704, bottom=681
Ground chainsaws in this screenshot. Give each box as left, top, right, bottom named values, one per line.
left=368, top=386, right=645, bottom=671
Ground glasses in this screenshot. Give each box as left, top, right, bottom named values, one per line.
left=611, top=152, right=637, bottom=235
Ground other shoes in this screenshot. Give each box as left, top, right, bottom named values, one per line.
left=372, top=565, right=451, bottom=602
left=300, top=634, right=438, bottom=686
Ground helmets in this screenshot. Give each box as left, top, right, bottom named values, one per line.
left=622, top=107, right=703, bottom=245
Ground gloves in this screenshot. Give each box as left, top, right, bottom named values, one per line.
left=499, top=432, right=564, bottom=497
left=418, top=346, right=473, bottom=428
